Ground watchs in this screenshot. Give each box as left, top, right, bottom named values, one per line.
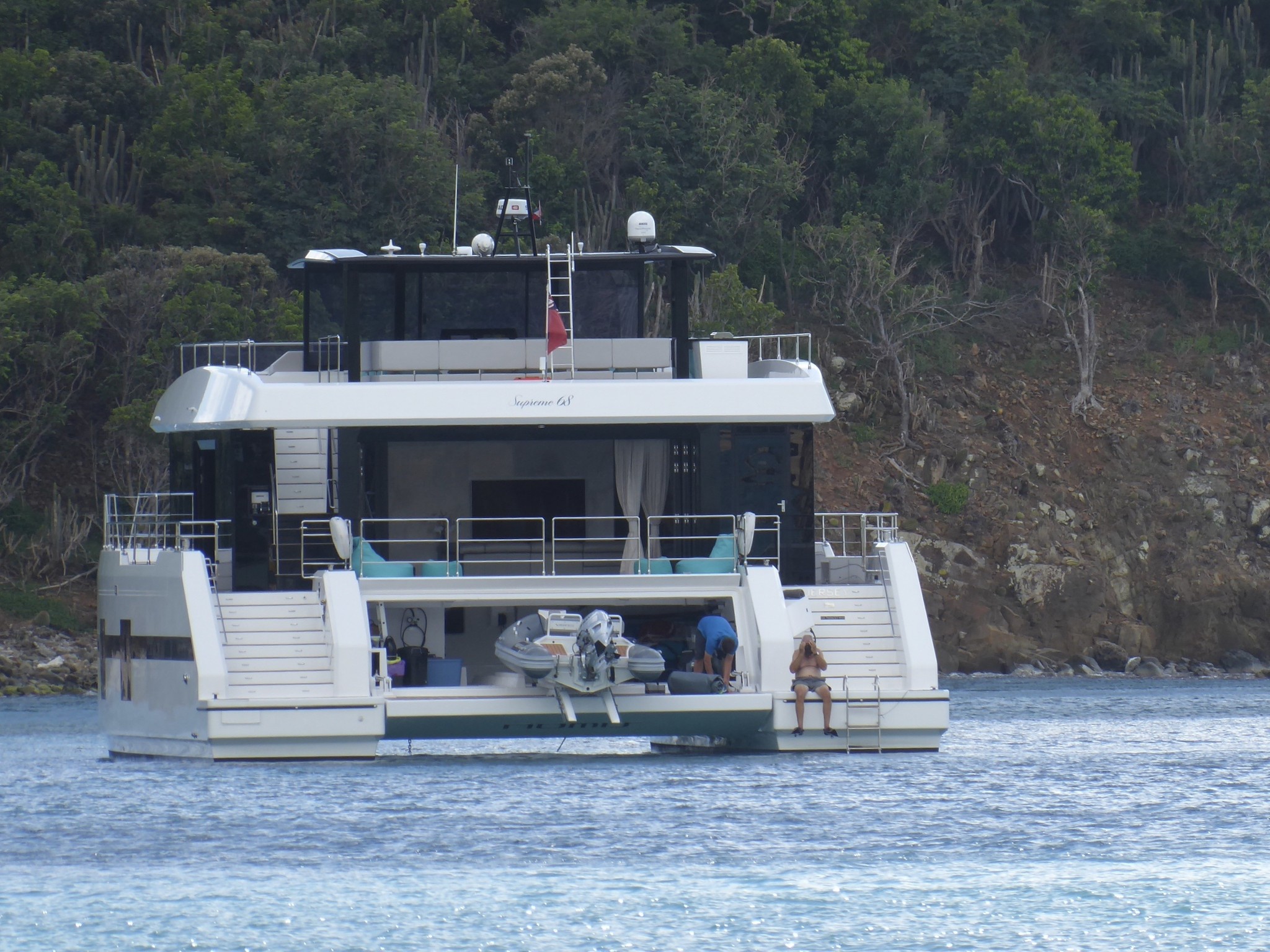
left=813, top=651, right=819, bottom=655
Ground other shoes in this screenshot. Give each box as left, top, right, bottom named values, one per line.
left=823, top=727, right=838, bottom=737
left=790, top=727, right=804, bottom=736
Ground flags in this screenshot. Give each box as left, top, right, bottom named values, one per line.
left=547, top=291, right=567, bottom=356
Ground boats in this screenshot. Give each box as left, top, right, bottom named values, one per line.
left=92, top=130, right=954, bottom=761
left=494, top=608, right=667, bottom=683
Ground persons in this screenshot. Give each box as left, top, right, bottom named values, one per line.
left=789, top=635, right=839, bottom=737
left=693, top=615, right=739, bottom=695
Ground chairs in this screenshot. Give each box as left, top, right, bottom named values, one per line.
left=675, top=534, right=739, bottom=574
left=351, top=537, right=414, bottom=579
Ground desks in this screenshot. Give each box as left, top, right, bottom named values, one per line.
left=634, top=556, right=673, bottom=574
left=421, top=559, right=462, bottom=577
